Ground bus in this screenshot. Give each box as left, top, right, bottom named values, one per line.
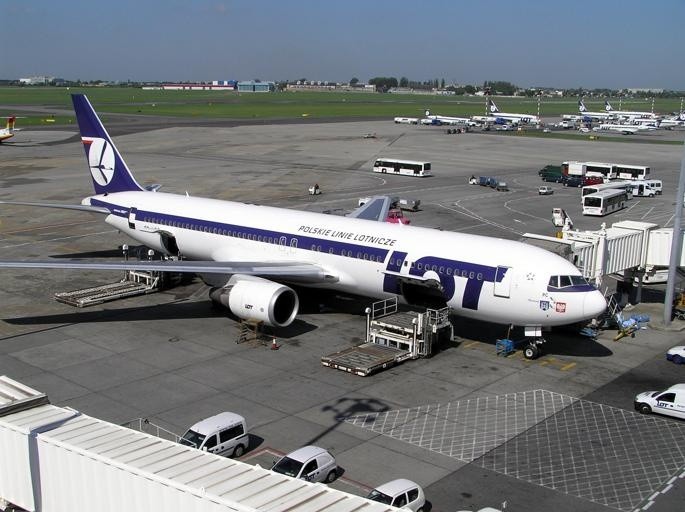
left=538, top=161, right=663, bottom=216
left=373, top=158, right=432, bottom=177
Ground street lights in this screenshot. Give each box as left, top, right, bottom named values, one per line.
left=619, top=94, right=625, bottom=110
left=581, top=94, right=587, bottom=101
left=484, top=86, right=492, bottom=126
left=535, top=89, right=543, bottom=130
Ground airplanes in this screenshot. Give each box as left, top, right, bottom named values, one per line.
left=0, top=92, right=607, bottom=359
left=0, top=116, right=27, bottom=140
left=489, top=95, right=685, bottom=136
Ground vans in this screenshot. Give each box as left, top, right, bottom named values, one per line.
left=178, top=411, right=249, bottom=456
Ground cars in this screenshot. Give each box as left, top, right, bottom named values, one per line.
left=468, top=176, right=507, bottom=191
left=270, top=446, right=337, bottom=482
left=634, top=383, right=685, bottom=419
left=666, top=346, right=685, bottom=364
left=366, top=478, right=426, bottom=512
